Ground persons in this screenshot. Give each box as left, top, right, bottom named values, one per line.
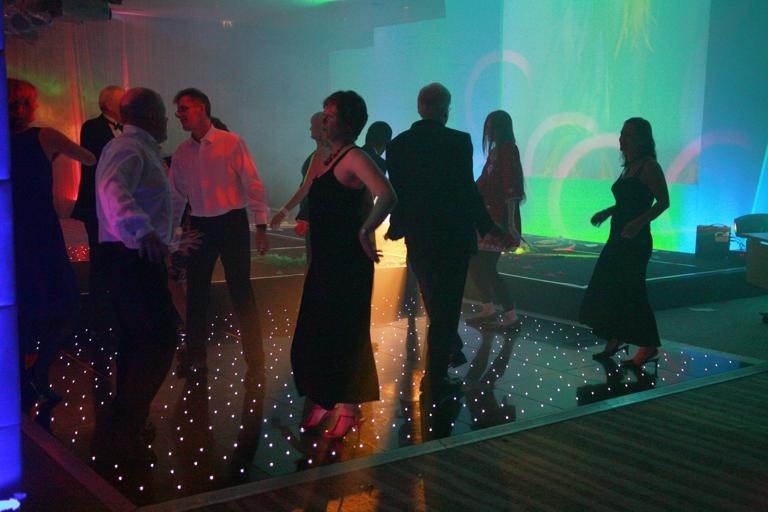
left=262, top=91, right=399, bottom=444
left=5, top=75, right=99, bottom=402
left=85, top=87, right=207, bottom=466
left=166, top=87, right=273, bottom=384
left=295, top=113, right=393, bottom=236
left=577, top=116, right=670, bottom=367
left=464, top=110, right=527, bottom=332
left=380, top=82, right=510, bottom=411
left=68, top=86, right=126, bottom=278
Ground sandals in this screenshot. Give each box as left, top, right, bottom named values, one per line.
left=466, top=312, right=522, bottom=330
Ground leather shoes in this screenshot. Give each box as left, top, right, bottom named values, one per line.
left=420, top=354, right=468, bottom=392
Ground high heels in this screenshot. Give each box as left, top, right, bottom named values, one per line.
left=299, top=403, right=365, bottom=439
left=620, top=357, right=664, bottom=373
left=592, top=344, right=630, bottom=361
